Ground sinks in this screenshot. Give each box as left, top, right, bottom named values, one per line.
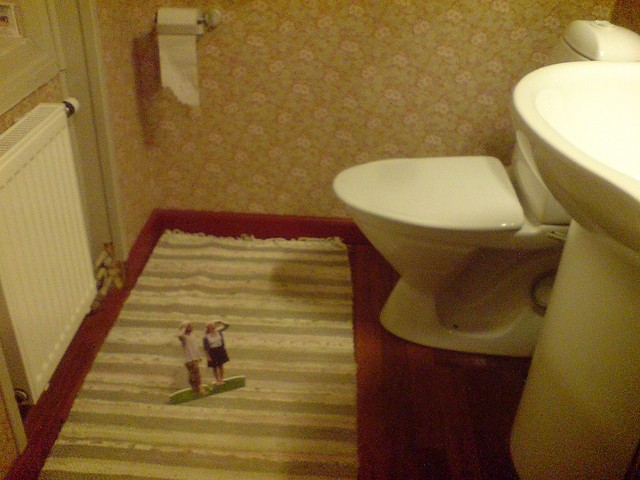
left=510, top=59, right=640, bottom=254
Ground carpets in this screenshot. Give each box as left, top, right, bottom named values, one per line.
left=39, top=230, right=359, bottom=479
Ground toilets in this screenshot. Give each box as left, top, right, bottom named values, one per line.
left=331, top=17, right=640, bottom=357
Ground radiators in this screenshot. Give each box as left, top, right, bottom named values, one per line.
left=0, top=97, right=98, bottom=404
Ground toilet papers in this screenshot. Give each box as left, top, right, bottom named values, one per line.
left=155, top=5, right=205, bottom=108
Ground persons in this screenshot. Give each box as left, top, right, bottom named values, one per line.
left=178, top=321, right=207, bottom=392
left=203, top=321, right=229, bottom=387
